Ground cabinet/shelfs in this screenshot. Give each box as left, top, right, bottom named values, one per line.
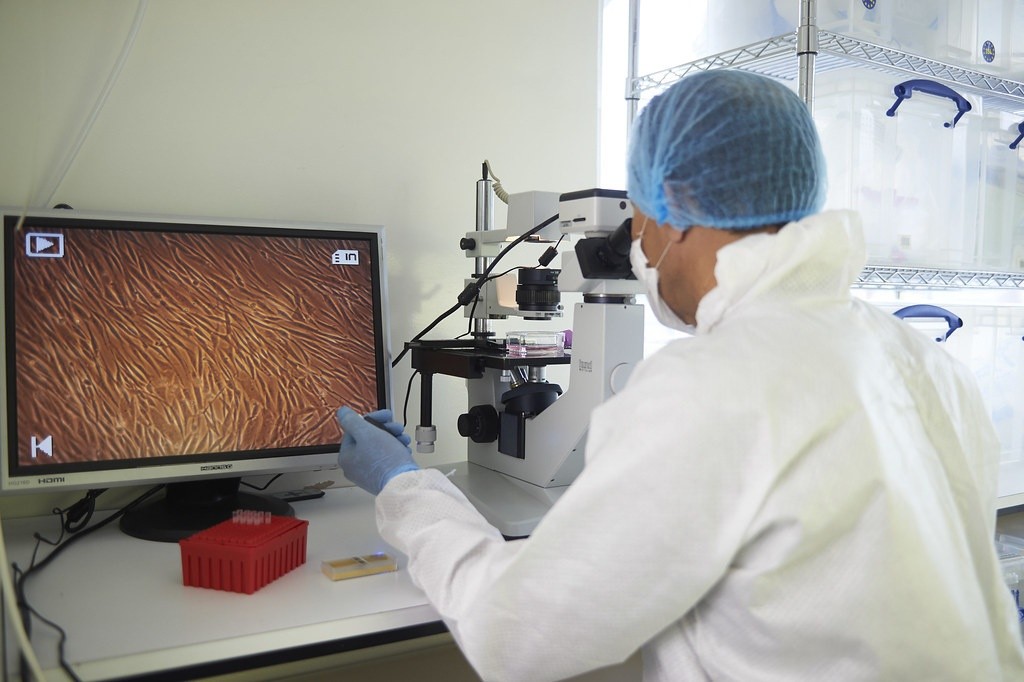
left=624, top=0, right=1024, bottom=517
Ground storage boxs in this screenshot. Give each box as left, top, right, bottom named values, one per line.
left=789, top=66, right=983, bottom=273
left=996, top=305, right=1024, bottom=498
left=978, top=0, right=1024, bottom=84
left=179, top=514, right=310, bottom=596
left=979, top=106, right=1024, bottom=275
left=872, top=301, right=997, bottom=424
left=704, top=0, right=978, bottom=70
left=995, top=532, right=1024, bottom=631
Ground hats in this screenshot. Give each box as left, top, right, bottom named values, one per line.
left=626, top=68, right=827, bottom=230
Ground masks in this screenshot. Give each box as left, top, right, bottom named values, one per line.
left=629, top=216, right=696, bottom=336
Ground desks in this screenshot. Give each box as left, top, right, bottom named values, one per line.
left=0, top=486, right=440, bottom=682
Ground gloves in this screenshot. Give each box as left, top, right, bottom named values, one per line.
left=337, top=406, right=419, bottom=496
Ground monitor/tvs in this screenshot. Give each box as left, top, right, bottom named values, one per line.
left=0, top=206, right=391, bottom=543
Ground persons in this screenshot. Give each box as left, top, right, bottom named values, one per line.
left=337, top=66, right=1024, bottom=681
